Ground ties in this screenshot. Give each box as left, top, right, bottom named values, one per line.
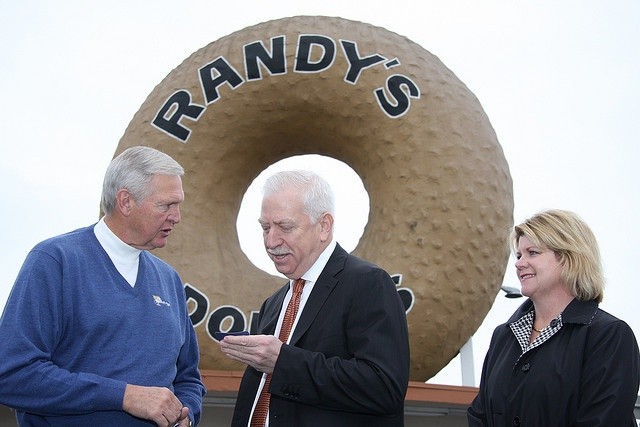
left=250, top=277, right=305, bottom=426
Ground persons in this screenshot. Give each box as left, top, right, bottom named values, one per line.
left=0, top=144, right=208, bottom=426
left=221, top=167, right=410, bottom=426
left=466, top=207, right=640, bottom=427
